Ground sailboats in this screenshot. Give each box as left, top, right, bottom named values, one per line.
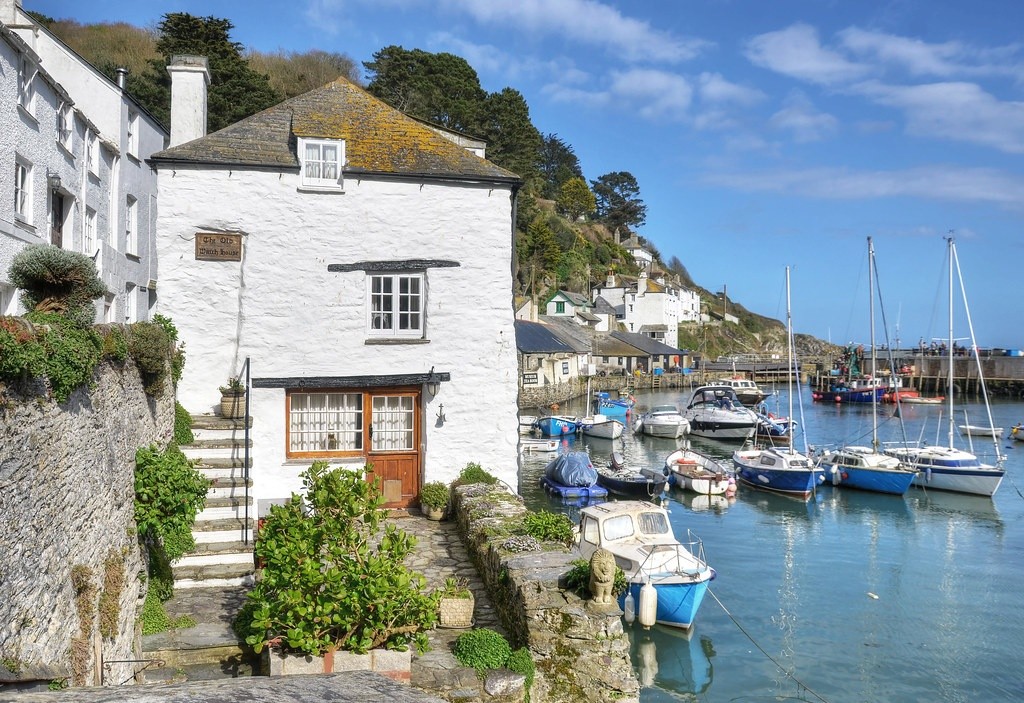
left=814, top=237, right=920, bottom=495
left=883, top=229, right=1007, bottom=501
left=731, top=265, right=824, bottom=497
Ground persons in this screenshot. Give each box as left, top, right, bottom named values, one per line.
left=720, top=391, right=732, bottom=410
left=932, top=342, right=937, bottom=356
left=953, top=341, right=957, bottom=356
left=923, top=342, right=928, bottom=356
left=940, top=342, right=945, bottom=356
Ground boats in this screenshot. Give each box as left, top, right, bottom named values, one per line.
left=638, top=404, right=690, bottom=441
left=745, top=408, right=796, bottom=439
left=682, top=385, right=758, bottom=439
left=540, top=475, right=609, bottom=498
left=618, top=613, right=713, bottom=703
left=593, top=452, right=668, bottom=499
left=567, top=499, right=714, bottom=631
left=519, top=410, right=577, bottom=437
left=520, top=438, right=560, bottom=452
left=1011, top=425, right=1024, bottom=441
left=579, top=414, right=624, bottom=440
left=591, top=387, right=635, bottom=417
left=666, top=485, right=728, bottom=512
left=958, top=425, right=1003, bottom=436
left=704, top=362, right=771, bottom=408
left=812, top=342, right=945, bottom=404
left=664, top=448, right=730, bottom=495
